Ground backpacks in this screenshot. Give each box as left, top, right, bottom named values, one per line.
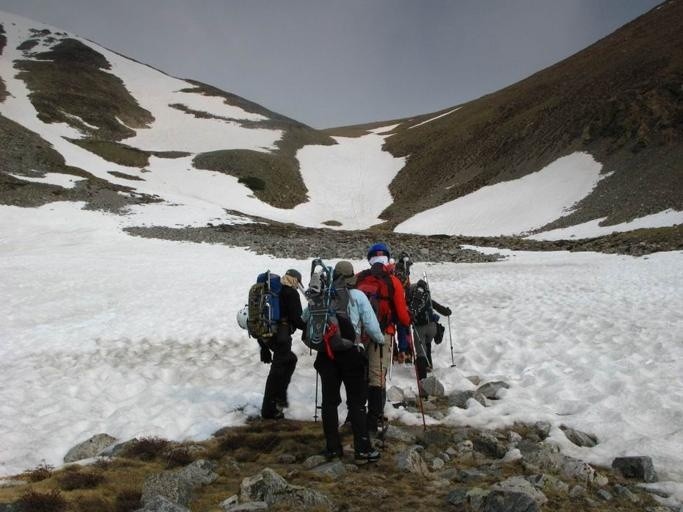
left=247, top=272, right=281, bottom=338
left=301, top=275, right=357, bottom=354
left=353, top=262, right=393, bottom=358
left=410, top=279, right=435, bottom=325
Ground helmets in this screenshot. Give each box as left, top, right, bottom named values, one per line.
left=236, top=306, right=250, bottom=330
left=366, top=244, right=389, bottom=259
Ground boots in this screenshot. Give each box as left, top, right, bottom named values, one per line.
left=367, top=387, right=387, bottom=423
left=425, top=342, right=431, bottom=373
left=413, top=357, right=427, bottom=400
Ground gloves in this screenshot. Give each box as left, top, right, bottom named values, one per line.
left=260, top=349, right=271, bottom=364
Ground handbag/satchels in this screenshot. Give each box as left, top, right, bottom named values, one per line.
left=433, top=323, right=444, bottom=344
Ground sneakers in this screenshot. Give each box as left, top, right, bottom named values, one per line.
left=354, top=447, right=381, bottom=465
left=261, top=408, right=285, bottom=419
left=275, top=394, right=288, bottom=407
left=326, top=444, right=343, bottom=459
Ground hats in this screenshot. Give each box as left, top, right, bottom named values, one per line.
left=333, top=261, right=356, bottom=287
left=286, top=269, right=305, bottom=289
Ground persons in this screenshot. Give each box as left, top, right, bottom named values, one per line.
left=403, top=278, right=452, bottom=401
left=389, top=250, right=410, bottom=365
left=257, top=267, right=308, bottom=421
left=296, top=259, right=385, bottom=467
left=340, top=243, right=410, bottom=438
